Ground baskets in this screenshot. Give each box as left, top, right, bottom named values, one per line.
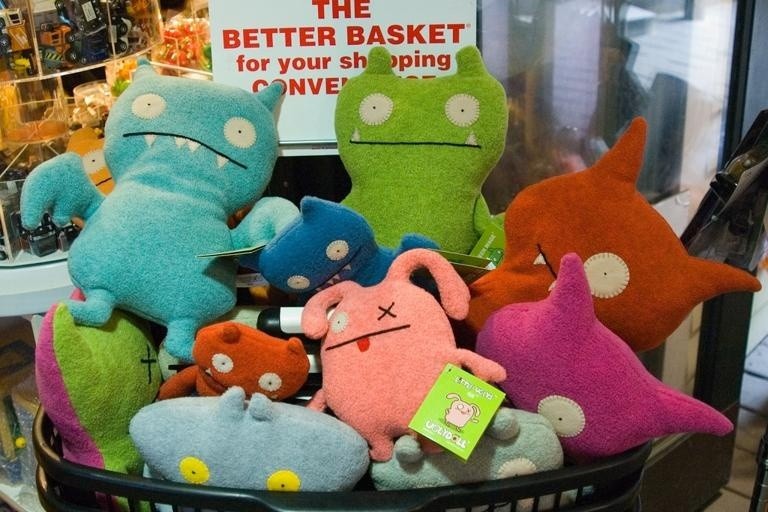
left=32, top=400, right=653, bottom=511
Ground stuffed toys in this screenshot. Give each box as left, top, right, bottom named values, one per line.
left=20, top=46, right=762, bottom=510
left=19, top=44, right=762, bottom=510
